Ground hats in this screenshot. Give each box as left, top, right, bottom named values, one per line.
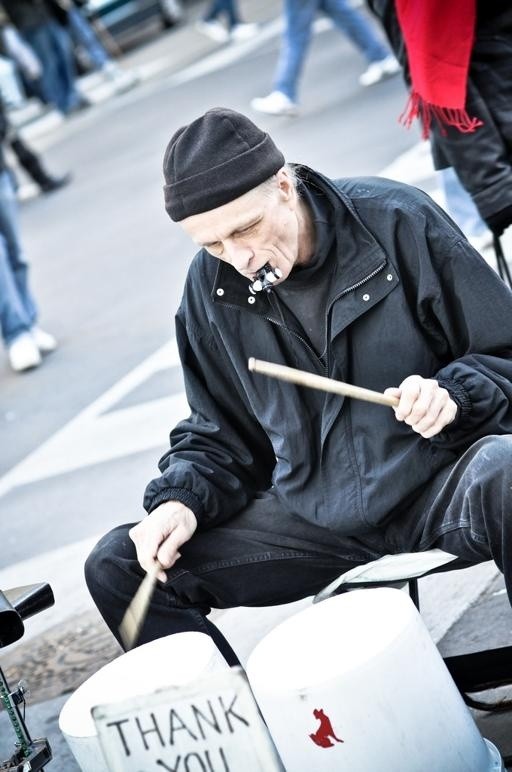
left=160, top=106, right=285, bottom=223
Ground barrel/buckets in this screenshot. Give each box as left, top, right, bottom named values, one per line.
left=243, top=587, right=502, bottom=771
left=56, top=630, right=229, bottom=772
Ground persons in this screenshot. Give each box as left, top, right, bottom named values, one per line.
left=82, top=108, right=512, bottom=675
left=193, top=2, right=261, bottom=57
left=1, top=97, right=69, bottom=372
left=248, top=1, right=406, bottom=118
left=1, top=0, right=110, bottom=118
left=367, top=0, right=512, bottom=228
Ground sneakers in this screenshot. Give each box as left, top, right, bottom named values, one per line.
left=251, top=91, right=297, bottom=117
left=27, top=325, right=57, bottom=355
left=358, top=54, right=402, bottom=87
left=8, top=332, right=41, bottom=373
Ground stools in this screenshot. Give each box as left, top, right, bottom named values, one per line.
left=310, top=555, right=512, bottom=712
